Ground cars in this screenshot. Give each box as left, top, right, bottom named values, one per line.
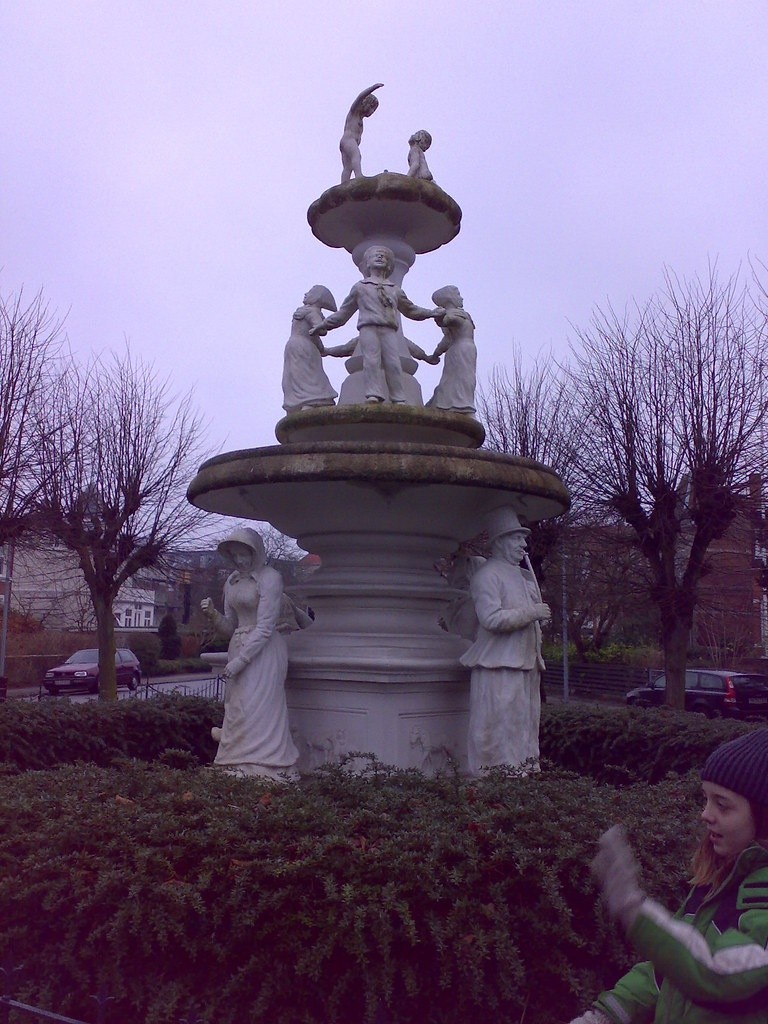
left=41, top=647, right=143, bottom=697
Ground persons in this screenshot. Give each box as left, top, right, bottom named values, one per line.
left=281, top=83, right=478, bottom=418
left=570, top=726, right=768, bottom=1024
left=449, top=505, right=551, bottom=779
left=201, top=528, right=313, bottom=785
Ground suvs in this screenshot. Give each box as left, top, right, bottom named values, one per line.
left=625, top=667, right=768, bottom=723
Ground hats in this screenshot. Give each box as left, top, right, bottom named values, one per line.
left=700, top=726, right=768, bottom=808
